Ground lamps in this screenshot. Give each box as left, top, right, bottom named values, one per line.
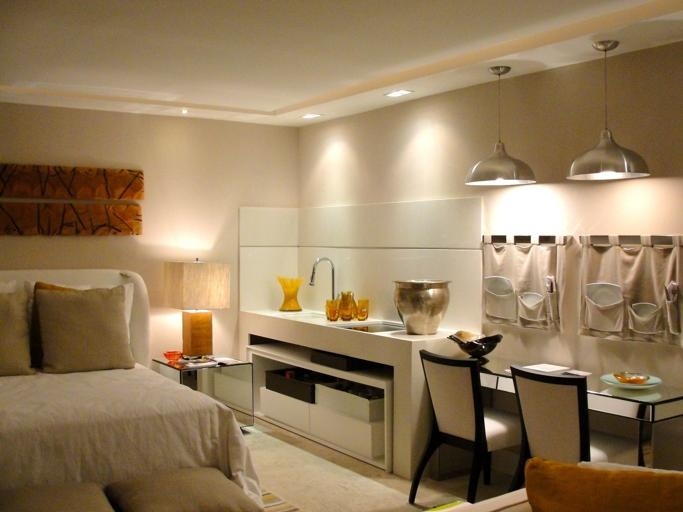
left=464, top=65, right=535, bottom=186
left=162, top=256, right=231, bottom=356
left=566, top=39, right=650, bottom=182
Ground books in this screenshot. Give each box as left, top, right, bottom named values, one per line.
left=185, top=361, right=219, bottom=369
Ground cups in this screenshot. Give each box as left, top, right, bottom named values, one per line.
left=326, top=291, right=369, bottom=321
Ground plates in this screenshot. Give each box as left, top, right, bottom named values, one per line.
left=601, top=373, right=663, bottom=389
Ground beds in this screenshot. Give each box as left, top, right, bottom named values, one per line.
left=0, top=363, right=264, bottom=509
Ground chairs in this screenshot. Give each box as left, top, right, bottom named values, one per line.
left=408, top=350, right=527, bottom=504
left=510, top=366, right=606, bottom=489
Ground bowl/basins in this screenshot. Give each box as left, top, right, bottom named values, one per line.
left=163, top=351, right=183, bottom=361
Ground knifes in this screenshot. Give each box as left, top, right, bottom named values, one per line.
left=663, top=283, right=671, bottom=301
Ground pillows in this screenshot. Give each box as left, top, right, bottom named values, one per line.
left=0, top=280, right=135, bottom=375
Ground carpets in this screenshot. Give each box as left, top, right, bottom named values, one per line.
left=259, top=487, right=302, bottom=512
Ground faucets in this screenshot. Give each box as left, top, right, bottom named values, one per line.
left=308, top=256, right=337, bottom=300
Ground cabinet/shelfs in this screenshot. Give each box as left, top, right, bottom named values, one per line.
left=246, top=312, right=392, bottom=473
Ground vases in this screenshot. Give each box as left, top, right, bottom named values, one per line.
left=276, top=278, right=304, bottom=313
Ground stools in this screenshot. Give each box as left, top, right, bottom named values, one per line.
left=0, top=465, right=264, bottom=512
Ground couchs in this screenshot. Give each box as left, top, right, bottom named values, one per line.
left=524, top=456, right=683, bottom=511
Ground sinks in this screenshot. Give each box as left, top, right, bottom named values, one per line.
left=332, top=319, right=405, bottom=333
